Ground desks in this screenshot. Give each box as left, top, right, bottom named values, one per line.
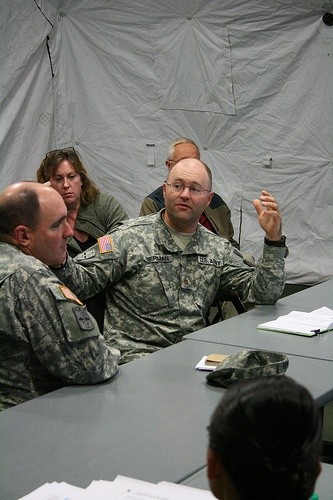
left=183, top=278, right=333, bottom=362
left=177, top=462, right=333, bottom=500
left=0, top=339, right=333, bottom=500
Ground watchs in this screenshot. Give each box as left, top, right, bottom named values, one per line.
left=263, top=235, right=287, bottom=248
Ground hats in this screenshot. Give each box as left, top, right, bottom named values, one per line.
left=205, top=349, right=289, bottom=389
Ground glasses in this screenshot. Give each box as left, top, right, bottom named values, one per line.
left=166, top=181, right=211, bottom=196
left=45, top=146, right=76, bottom=160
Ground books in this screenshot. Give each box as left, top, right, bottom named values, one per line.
left=258, top=305, right=333, bottom=337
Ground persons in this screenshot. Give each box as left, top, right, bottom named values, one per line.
left=50, top=157, right=288, bottom=366
left=206, top=376, right=323, bottom=500
left=36, top=146, right=130, bottom=335
left=0, top=181, right=123, bottom=412
left=140, top=138, right=247, bottom=314
